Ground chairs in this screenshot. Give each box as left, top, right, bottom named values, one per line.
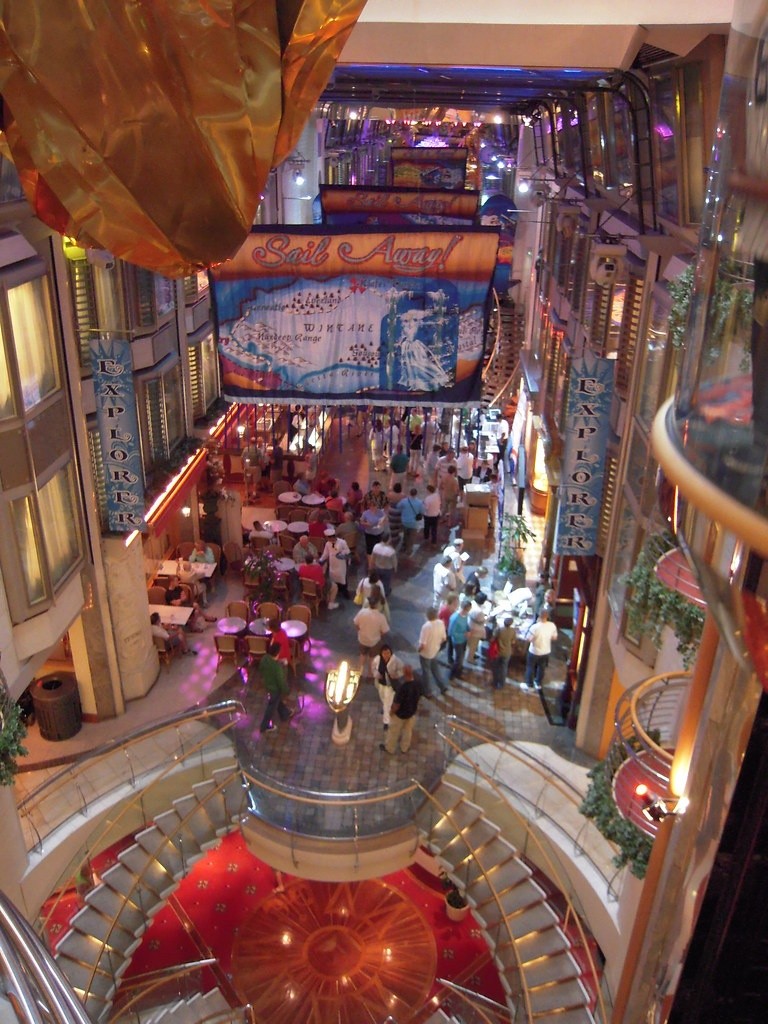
left=214, top=634, right=299, bottom=675
left=275, top=505, right=339, bottom=522
left=153, top=632, right=180, bottom=664
left=148, top=583, right=198, bottom=604
left=246, top=535, right=326, bottom=552
left=227, top=602, right=311, bottom=647
left=176, top=542, right=221, bottom=576
left=242, top=568, right=319, bottom=619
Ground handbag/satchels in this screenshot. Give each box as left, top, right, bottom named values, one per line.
left=353, top=578, right=365, bottom=604
left=415, top=513, right=423, bottom=520
left=489, top=642, right=500, bottom=658
left=391, top=679, right=401, bottom=691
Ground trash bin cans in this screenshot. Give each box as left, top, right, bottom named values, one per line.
left=30, top=675, right=82, bottom=742
left=463, top=482, right=490, bottom=531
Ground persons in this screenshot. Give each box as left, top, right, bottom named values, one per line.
left=150, top=611, right=198, bottom=656
left=188, top=540, right=222, bottom=593
left=229, top=405, right=509, bottom=610
left=439, top=589, right=558, bottom=692
left=206, top=453, right=225, bottom=490
left=379, top=664, right=423, bottom=755
left=417, top=606, right=449, bottom=696
left=259, top=646, right=295, bottom=732
left=178, top=561, right=211, bottom=608
left=263, top=616, right=292, bottom=678
left=370, top=644, right=405, bottom=732
left=234, top=630, right=250, bottom=669
left=164, top=575, right=217, bottom=633
left=353, top=594, right=391, bottom=679
left=535, top=249, right=543, bottom=282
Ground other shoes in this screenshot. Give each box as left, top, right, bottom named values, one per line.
left=266, top=725, right=276, bottom=733
left=383, top=723, right=388, bottom=730
left=422, top=691, right=432, bottom=698
left=184, top=648, right=197, bottom=656
left=520, top=682, right=534, bottom=692
left=288, top=709, right=295, bottom=718
left=327, top=602, right=339, bottom=610
left=204, top=615, right=217, bottom=622
left=440, top=687, right=448, bottom=695
left=190, top=626, right=203, bottom=633
left=534, top=681, right=542, bottom=689
left=379, top=743, right=386, bottom=750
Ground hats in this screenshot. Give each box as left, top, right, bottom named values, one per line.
left=324, top=528, right=335, bottom=536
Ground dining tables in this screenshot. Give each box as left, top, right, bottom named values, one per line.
left=279, top=492, right=347, bottom=505
left=245, top=557, right=319, bottom=572
left=150, top=604, right=194, bottom=625
left=263, top=520, right=335, bottom=533
left=158, top=560, right=217, bottom=577
left=217, top=616, right=307, bottom=639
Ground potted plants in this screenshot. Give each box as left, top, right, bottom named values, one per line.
left=441, top=868, right=470, bottom=921
left=492, top=512, right=536, bottom=592
